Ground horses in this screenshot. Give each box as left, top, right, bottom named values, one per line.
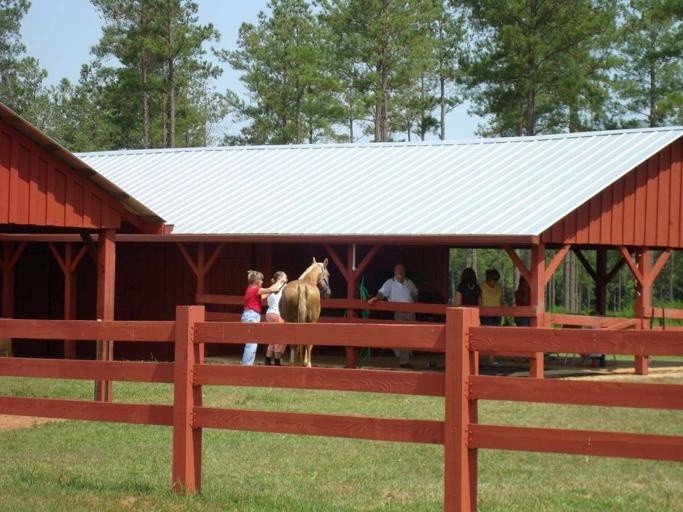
left=278, top=256, right=331, bottom=368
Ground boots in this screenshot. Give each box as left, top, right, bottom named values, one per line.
left=264, top=357, right=271, bottom=364
left=274, top=359, right=280, bottom=365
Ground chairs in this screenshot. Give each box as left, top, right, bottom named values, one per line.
left=561, top=324, right=583, bottom=366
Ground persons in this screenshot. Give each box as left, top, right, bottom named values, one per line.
left=454, top=268, right=483, bottom=307
left=367, top=264, right=419, bottom=369
left=265, top=271, right=287, bottom=366
left=514, top=276, right=531, bottom=327
left=580, top=311, right=606, bottom=367
left=241, top=270, right=285, bottom=366
left=479, top=269, right=514, bottom=368
